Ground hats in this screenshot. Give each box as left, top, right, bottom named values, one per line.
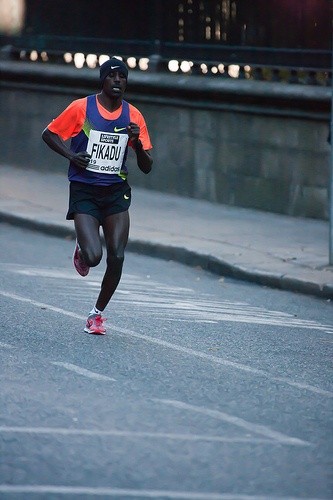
left=99, top=58, right=129, bottom=84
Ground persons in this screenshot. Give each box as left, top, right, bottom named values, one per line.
left=41, top=59, right=153, bottom=335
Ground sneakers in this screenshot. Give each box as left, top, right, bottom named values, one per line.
left=84, top=313, right=107, bottom=336
left=73, top=236, right=89, bottom=277
